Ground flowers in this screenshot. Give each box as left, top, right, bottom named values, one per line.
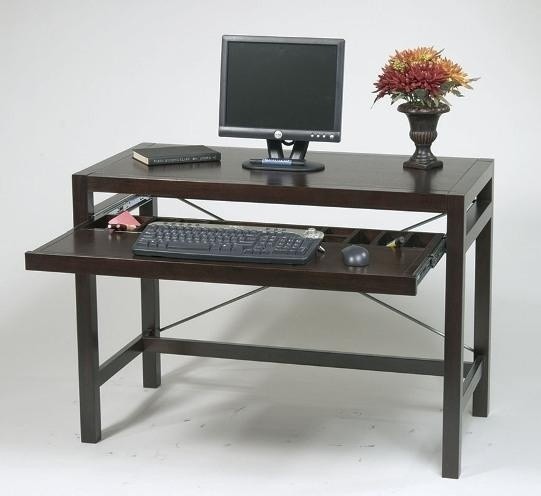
left=370, top=44, right=482, bottom=109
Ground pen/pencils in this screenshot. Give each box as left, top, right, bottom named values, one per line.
left=386, top=236, right=405, bottom=247
left=249, top=158, right=305, bottom=166
left=318, top=245, right=326, bottom=252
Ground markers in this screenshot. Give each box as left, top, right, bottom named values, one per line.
left=107, top=224, right=140, bottom=231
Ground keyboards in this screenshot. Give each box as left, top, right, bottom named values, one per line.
left=131, top=221, right=325, bottom=265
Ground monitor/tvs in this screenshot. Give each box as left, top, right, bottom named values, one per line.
left=218, top=35, right=345, bottom=172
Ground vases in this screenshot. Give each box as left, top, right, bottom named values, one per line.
left=397, top=102, right=450, bottom=170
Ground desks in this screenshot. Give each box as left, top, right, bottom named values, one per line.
left=26, top=144, right=495, bottom=478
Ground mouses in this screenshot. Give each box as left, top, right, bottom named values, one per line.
left=341, top=245, right=370, bottom=267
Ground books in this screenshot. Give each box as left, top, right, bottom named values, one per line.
left=132, top=144, right=221, bottom=165
